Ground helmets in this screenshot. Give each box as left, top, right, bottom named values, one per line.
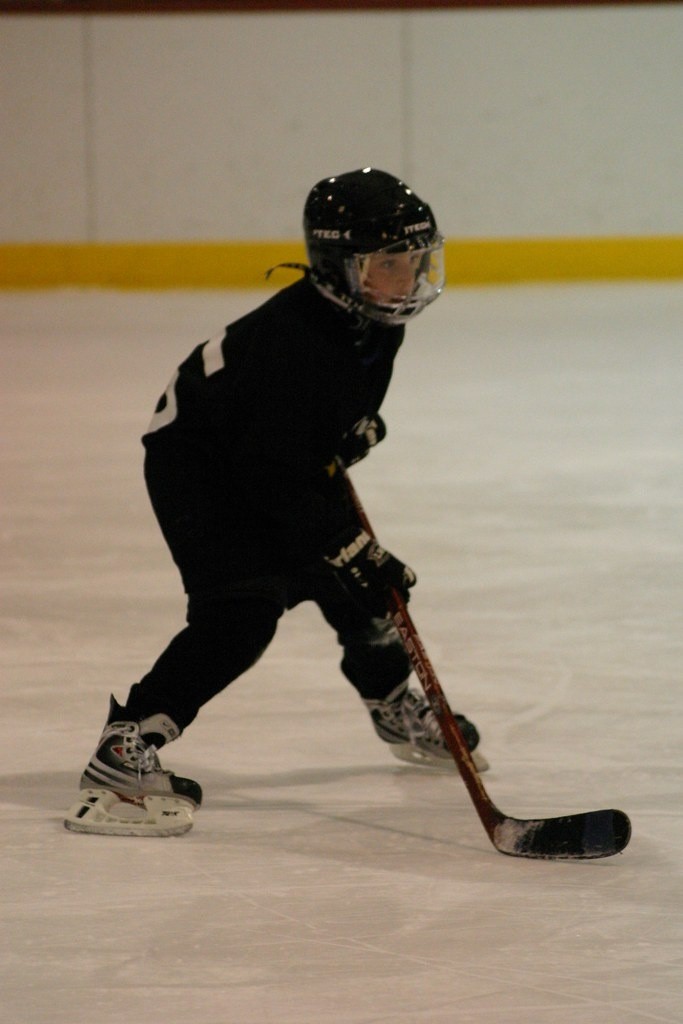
left=303, top=168, right=444, bottom=323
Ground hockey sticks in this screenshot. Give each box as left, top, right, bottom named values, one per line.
left=342, top=467, right=635, bottom=859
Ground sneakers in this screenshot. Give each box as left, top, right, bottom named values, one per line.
left=64, top=695, right=202, bottom=836
left=361, top=678, right=490, bottom=771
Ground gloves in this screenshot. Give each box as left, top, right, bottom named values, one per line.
left=325, top=527, right=417, bottom=621
left=329, top=412, right=386, bottom=467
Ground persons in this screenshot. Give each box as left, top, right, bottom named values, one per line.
left=80, top=167, right=481, bottom=812
